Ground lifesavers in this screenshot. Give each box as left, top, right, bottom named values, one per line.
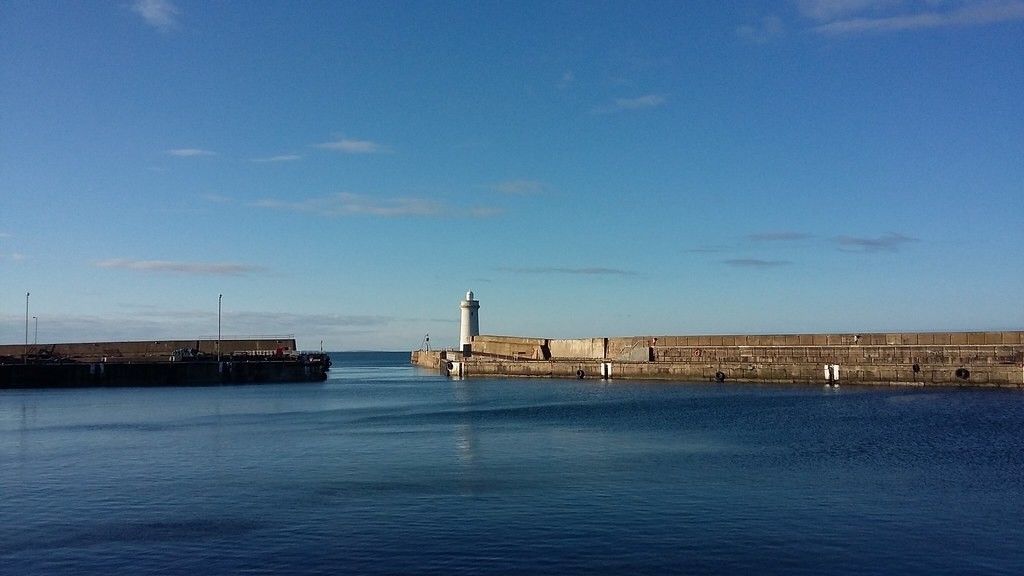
left=694, top=349, right=701, bottom=356
left=447, top=363, right=453, bottom=369
left=576, top=369, right=583, bottom=377
left=714, top=371, right=725, bottom=381
left=956, top=368, right=969, bottom=379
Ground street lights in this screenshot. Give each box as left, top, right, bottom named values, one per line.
left=34, top=316, right=37, bottom=346
left=25, top=292, right=30, bottom=362
left=217, top=293, right=222, bottom=360
